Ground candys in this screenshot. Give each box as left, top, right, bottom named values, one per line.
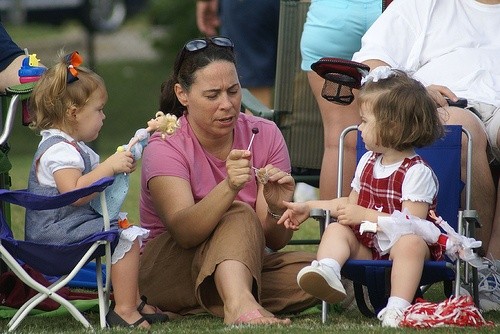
left=258, top=168, right=266, bottom=177
left=117, top=146, right=124, bottom=154
left=250, top=127, right=260, bottom=134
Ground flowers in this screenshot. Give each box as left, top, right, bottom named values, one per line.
left=360, top=65, right=396, bottom=87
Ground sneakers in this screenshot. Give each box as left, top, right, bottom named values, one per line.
left=478, top=259, right=500, bottom=315
left=376, top=303, right=405, bottom=329
left=296, top=259, right=347, bottom=304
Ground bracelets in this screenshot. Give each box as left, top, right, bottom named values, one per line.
left=267, top=208, right=282, bottom=220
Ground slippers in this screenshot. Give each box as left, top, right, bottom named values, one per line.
left=232, top=308, right=275, bottom=326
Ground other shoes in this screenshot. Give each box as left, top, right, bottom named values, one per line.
left=105, top=308, right=153, bottom=330
left=137, top=296, right=169, bottom=323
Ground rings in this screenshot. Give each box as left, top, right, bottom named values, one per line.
left=287, top=172, right=291, bottom=176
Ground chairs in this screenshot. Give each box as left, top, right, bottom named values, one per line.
left=0, top=84, right=125, bottom=334
left=239, top=0, right=325, bottom=246
left=309, top=123, right=483, bottom=324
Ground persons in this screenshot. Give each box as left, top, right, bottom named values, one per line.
left=138, top=36, right=353, bottom=330
left=300, top=0, right=391, bottom=317
left=276, top=66, right=446, bottom=329
left=90, top=110, right=179, bottom=221
left=0, top=21, right=49, bottom=96
left=196, top=0, right=281, bottom=120
left=26, top=51, right=170, bottom=334
left=358, top=209, right=485, bottom=270
left=350, top=0, right=500, bottom=315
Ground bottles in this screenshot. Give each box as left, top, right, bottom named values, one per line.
left=18, top=56, right=47, bottom=126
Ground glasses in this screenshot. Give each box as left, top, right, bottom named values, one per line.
left=174, top=36, right=235, bottom=76
